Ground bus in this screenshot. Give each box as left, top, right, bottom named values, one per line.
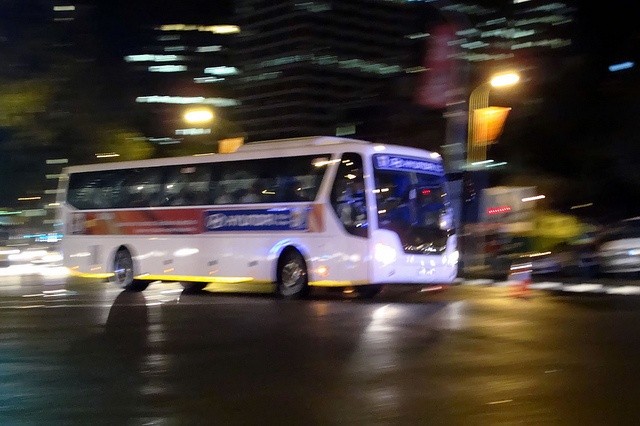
left=56, top=136, right=460, bottom=300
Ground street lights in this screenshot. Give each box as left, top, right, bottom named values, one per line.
left=171, top=103, right=249, bottom=150
left=464, top=67, right=524, bottom=286
